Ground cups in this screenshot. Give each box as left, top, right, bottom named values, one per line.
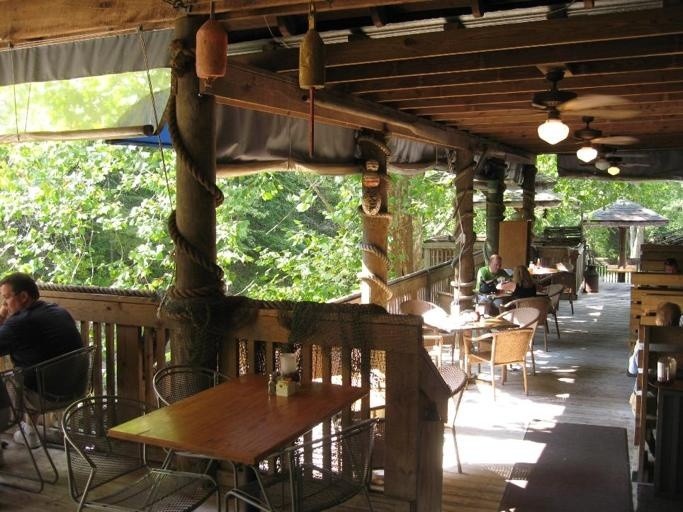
left=279, top=354, right=301, bottom=386
left=501, top=278, right=505, bottom=288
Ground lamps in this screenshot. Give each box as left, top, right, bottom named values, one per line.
left=532, top=69, right=577, bottom=146
left=568, top=114, right=652, bottom=177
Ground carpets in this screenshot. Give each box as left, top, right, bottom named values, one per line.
left=497, top=416, right=635, bottom=512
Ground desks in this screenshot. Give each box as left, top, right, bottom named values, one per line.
left=314, top=365, right=385, bottom=478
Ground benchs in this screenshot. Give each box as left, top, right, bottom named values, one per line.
left=628, top=243, right=682, bottom=512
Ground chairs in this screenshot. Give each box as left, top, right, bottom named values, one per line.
left=0, top=344, right=101, bottom=494
left=61, top=395, right=222, bottom=512
left=437, top=263, right=577, bottom=351
left=398, top=299, right=540, bottom=398
left=225, top=417, right=376, bottom=510
left=419, top=364, right=467, bottom=476
left=137, top=365, right=298, bottom=509
left=0, top=371, right=43, bottom=496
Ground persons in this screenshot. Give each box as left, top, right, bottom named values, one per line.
left=629, top=302, right=683, bottom=484
left=0, top=273, right=89, bottom=450
left=664, top=258, right=679, bottom=275
left=475, top=254, right=537, bottom=372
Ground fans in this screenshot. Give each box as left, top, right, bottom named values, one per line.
left=532, top=89, right=642, bottom=120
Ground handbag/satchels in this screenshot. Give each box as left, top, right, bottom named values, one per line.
left=480, top=279, right=497, bottom=293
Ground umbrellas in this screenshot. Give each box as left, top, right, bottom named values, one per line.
left=105, top=86, right=172, bottom=147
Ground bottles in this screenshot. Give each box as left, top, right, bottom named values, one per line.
left=266, top=373, right=276, bottom=395
left=528, top=256, right=541, bottom=273
left=655, top=350, right=677, bottom=384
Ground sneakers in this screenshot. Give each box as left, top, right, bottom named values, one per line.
left=13, top=423, right=41, bottom=448
